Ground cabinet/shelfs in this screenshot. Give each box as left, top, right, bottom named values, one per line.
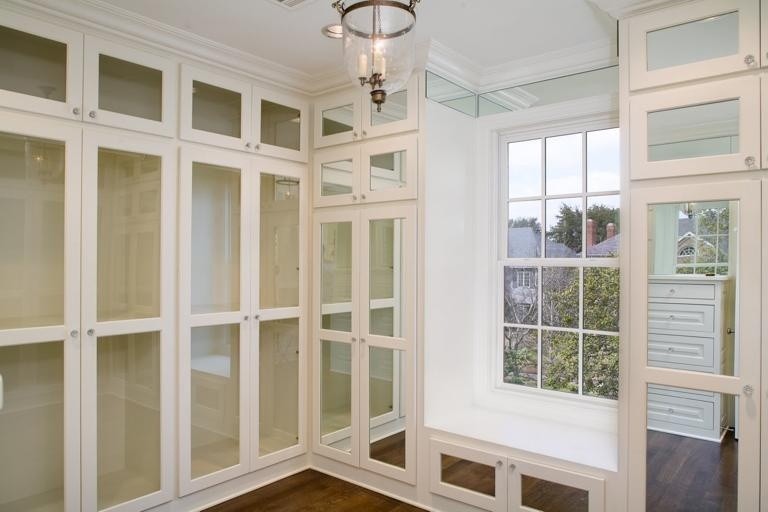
left=114, top=184, right=159, bottom=222
left=1, top=177, right=65, bottom=412
left=103, top=225, right=159, bottom=411
left=645, top=271, right=736, bottom=442
left=327, top=266, right=394, bottom=382
left=189, top=363, right=232, bottom=439
left=115, top=155, right=161, bottom=183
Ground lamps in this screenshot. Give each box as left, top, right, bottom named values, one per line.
left=330, top=0, right=422, bottom=114
left=274, top=178, right=298, bottom=203
left=25, top=85, right=64, bottom=187
